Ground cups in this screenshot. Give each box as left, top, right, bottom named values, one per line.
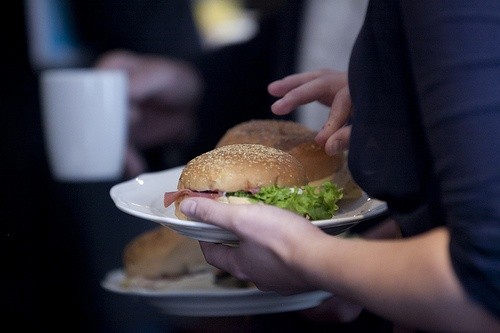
left=39, top=68, right=127, bottom=182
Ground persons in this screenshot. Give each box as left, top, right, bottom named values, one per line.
left=86, top=0, right=368, bottom=333
left=179, top=0, right=500, bottom=333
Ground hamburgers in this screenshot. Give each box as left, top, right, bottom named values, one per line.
left=164, top=143, right=344, bottom=223
left=216, top=120, right=362, bottom=199
left=122, top=227, right=228, bottom=289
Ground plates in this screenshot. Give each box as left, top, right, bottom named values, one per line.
left=101, top=269, right=337, bottom=316
left=108, top=165, right=389, bottom=243
